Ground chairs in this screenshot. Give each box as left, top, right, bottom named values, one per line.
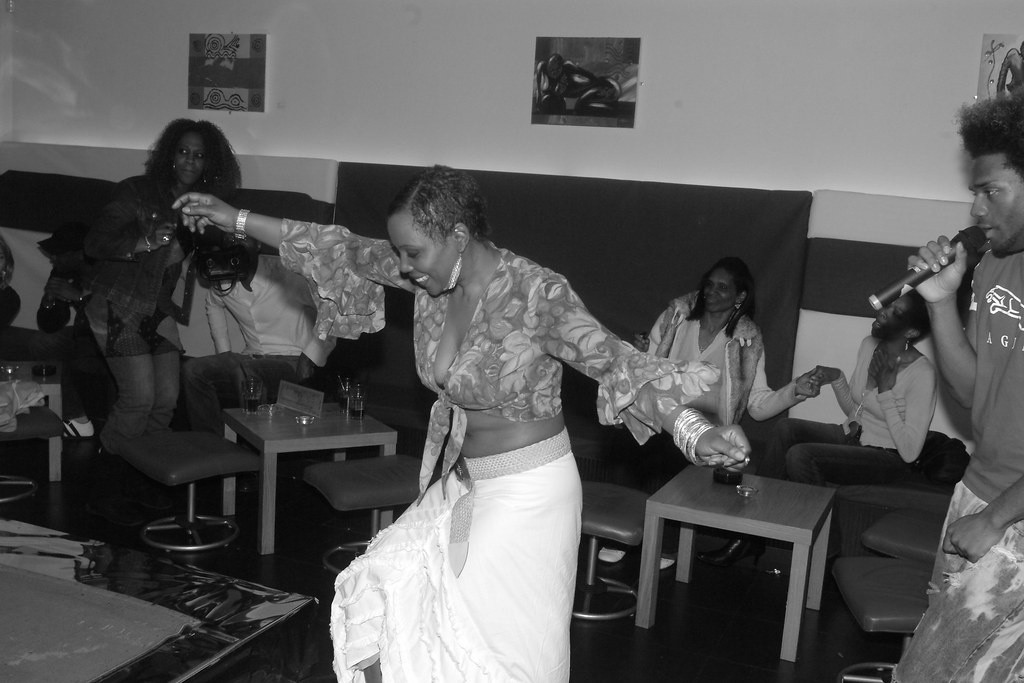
left=0, top=161, right=958, bottom=488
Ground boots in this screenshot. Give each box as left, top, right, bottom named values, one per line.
left=140, top=469, right=184, bottom=520
left=83, top=452, right=144, bottom=525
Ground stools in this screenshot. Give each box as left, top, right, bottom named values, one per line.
left=116, top=430, right=262, bottom=565
left=834, top=553, right=936, bottom=683
left=573, top=482, right=651, bottom=621
left=0, top=408, right=62, bottom=507
left=864, top=507, right=945, bottom=561
left=303, top=456, right=439, bottom=573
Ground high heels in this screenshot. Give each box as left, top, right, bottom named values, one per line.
left=767, top=551, right=797, bottom=575
left=696, top=534, right=765, bottom=568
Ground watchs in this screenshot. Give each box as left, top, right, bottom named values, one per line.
left=233, top=208, right=252, bottom=240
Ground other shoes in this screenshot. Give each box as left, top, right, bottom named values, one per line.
left=659, top=552, right=682, bottom=569
left=598, top=541, right=627, bottom=562
left=235, top=469, right=261, bottom=493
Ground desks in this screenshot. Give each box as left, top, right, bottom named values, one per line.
left=0, top=361, right=63, bottom=481
left=222, top=404, right=397, bottom=555
left=636, top=464, right=837, bottom=661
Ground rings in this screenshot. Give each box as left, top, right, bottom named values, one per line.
left=745, top=455, right=750, bottom=467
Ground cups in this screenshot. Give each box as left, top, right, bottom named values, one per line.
left=337, top=378, right=367, bottom=421
left=242, top=378, right=263, bottom=415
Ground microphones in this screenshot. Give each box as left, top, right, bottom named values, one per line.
left=868, top=225, right=987, bottom=311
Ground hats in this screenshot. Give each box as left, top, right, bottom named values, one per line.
left=37, top=222, right=90, bottom=254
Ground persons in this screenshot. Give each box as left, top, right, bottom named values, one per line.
left=777, top=89, right=1024, bottom=683
left=0, top=235, right=21, bottom=329
left=596, top=257, right=821, bottom=570
left=168, top=165, right=751, bottom=683
left=37, top=118, right=337, bottom=525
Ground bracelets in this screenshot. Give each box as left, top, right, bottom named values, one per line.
left=673, top=408, right=716, bottom=466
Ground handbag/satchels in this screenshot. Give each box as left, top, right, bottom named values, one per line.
left=196, top=245, right=249, bottom=280
left=911, top=429, right=971, bottom=484
